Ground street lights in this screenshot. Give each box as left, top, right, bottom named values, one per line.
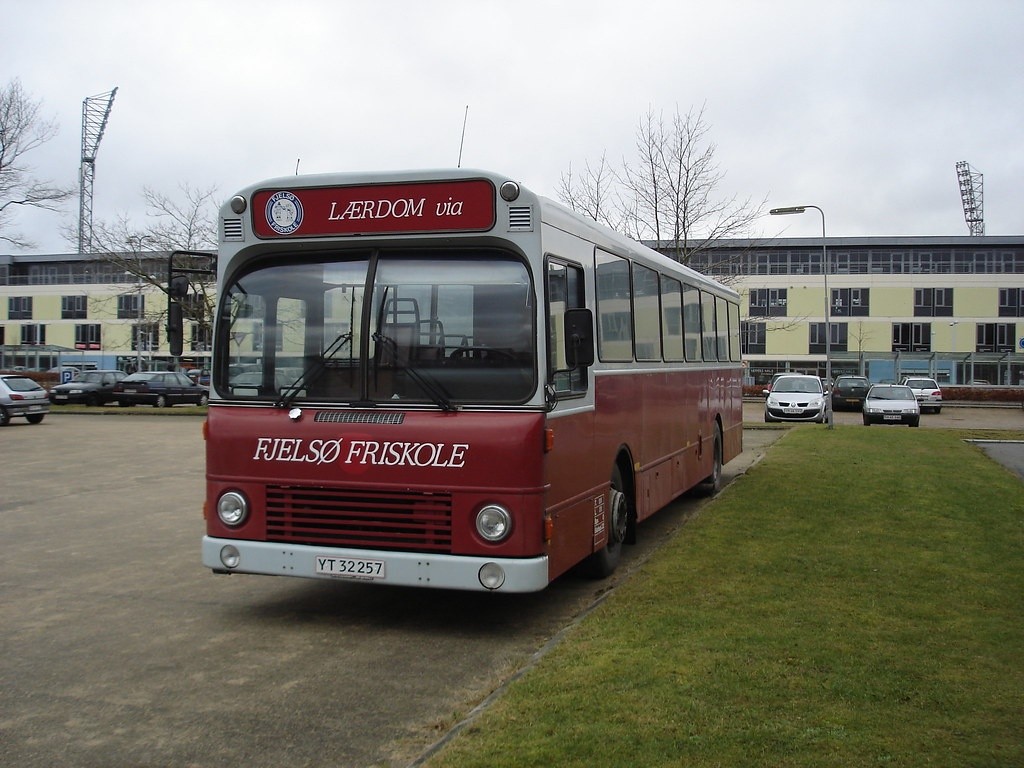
left=769, top=204, right=834, bottom=429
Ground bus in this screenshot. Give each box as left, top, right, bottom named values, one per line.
left=164, top=169, right=743, bottom=595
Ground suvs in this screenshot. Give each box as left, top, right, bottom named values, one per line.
left=895, top=375, right=943, bottom=414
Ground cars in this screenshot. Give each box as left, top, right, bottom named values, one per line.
left=111, top=371, right=207, bottom=408
left=231, top=364, right=305, bottom=398
left=48, top=370, right=129, bottom=406
left=831, top=375, right=870, bottom=411
left=0, top=374, right=51, bottom=428
left=770, top=372, right=805, bottom=387
left=821, top=378, right=835, bottom=389
left=762, top=375, right=829, bottom=424
left=186, top=368, right=211, bottom=386
left=859, top=384, right=920, bottom=427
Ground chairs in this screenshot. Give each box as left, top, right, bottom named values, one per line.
left=841, top=381, right=848, bottom=388
left=857, top=381, right=864, bottom=387
left=805, top=382, right=816, bottom=389
left=897, top=392, right=907, bottom=400
left=782, top=382, right=793, bottom=390
